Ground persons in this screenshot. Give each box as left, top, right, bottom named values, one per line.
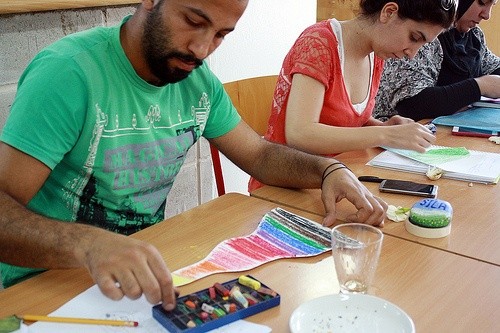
left=371, top=0, right=500, bottom=120
left=0, top=0, right=389, bottom=311
left=248, top=0, right=457, bottom=193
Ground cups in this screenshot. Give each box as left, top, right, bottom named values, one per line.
left=331, top=223, right=384, bottom=294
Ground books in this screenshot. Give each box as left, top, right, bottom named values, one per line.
left=365, top=145, right=500, bottom=185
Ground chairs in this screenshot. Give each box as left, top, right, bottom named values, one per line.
left=210, top=75, right=281, bottom=196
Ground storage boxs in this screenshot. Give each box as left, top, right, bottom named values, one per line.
left=152, top=275, right=280, bottom=333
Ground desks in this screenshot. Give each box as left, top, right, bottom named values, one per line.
left=251, top=105, right=500, bottom=265
left=0, top=192, right=500, bottom=333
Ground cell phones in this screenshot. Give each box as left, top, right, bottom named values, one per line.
left=452, top=126, right=498, bottom=138
left=378, top=180, right=438, bottom=198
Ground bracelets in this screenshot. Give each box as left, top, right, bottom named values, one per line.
left=320, top=162, right=353, bottom=190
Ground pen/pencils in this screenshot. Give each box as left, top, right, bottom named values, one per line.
left=357, top=174, right=385, bottom=183
left=12, top=313, right=139, bottom=328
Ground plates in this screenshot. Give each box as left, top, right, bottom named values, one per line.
left=289, top=293, right=415, bottom=333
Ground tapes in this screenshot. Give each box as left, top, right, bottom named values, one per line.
left=404, top=215, right=452, bottom=240
